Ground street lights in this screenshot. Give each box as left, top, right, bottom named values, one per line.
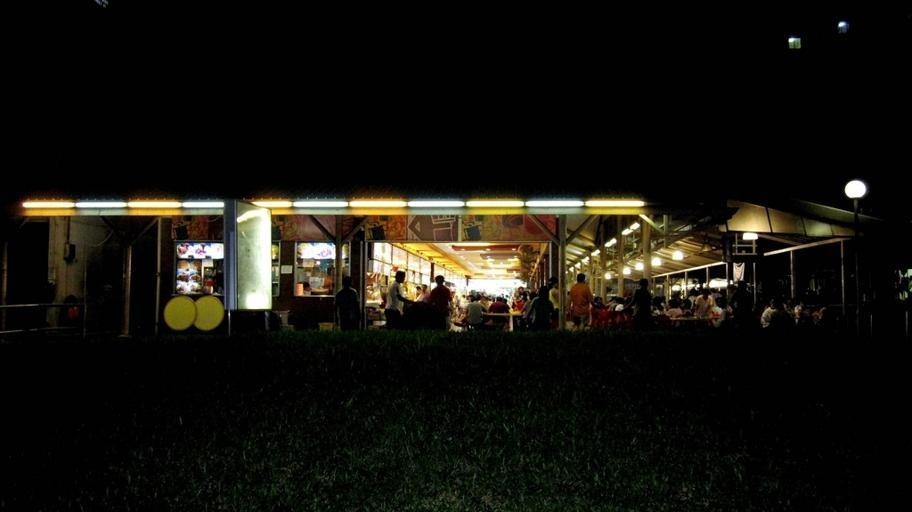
left=843, top=179, right=868, bottom=321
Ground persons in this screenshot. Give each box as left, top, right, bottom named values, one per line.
left=381, top=271, right=560, bottom=333
left=334, top=276, right=364, bottom=331
left=566, top=275, right=828, bottom=330
left=324, top=265, right=336, bottom=295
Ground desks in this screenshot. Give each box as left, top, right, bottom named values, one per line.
left=467, top=311, right=526, bottom=331
